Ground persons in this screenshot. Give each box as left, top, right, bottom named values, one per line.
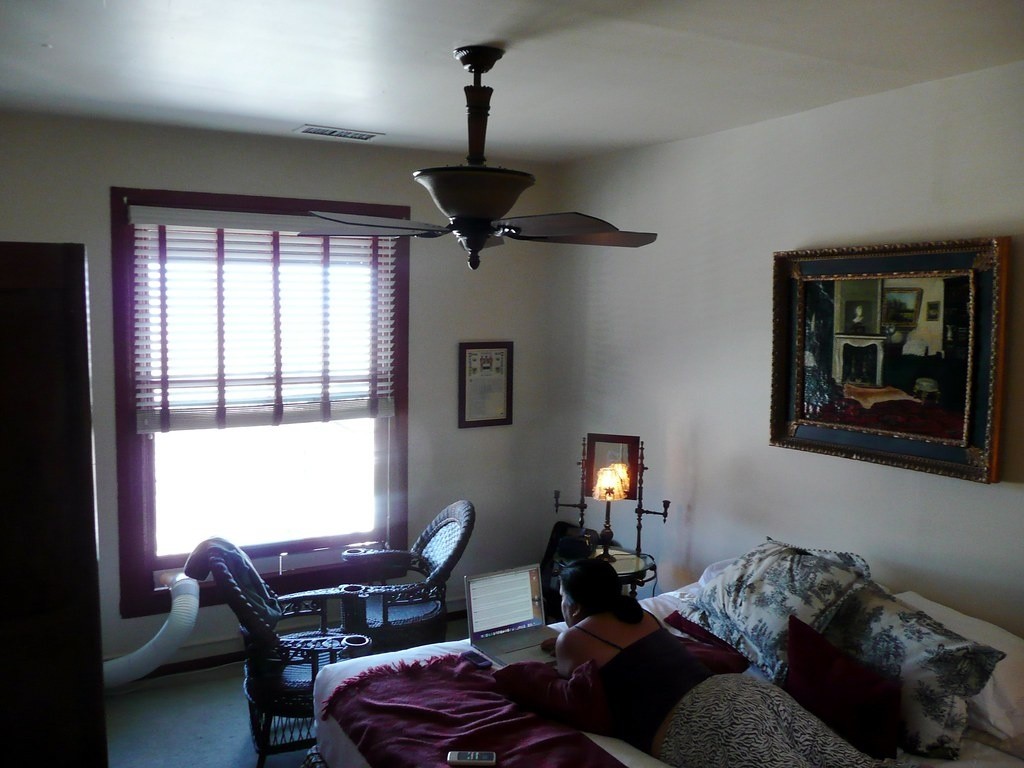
left=540, top=559, right=935, bottom=768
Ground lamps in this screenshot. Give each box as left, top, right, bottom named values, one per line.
left=591, top=467, right=627, bottom=564
left=611, top=462, right=630, bottom=492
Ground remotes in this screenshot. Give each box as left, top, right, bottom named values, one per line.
left=447, top=751, right=496, bottom=766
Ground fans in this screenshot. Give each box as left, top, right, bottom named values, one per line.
left=296, top=44, right=658, bottom=270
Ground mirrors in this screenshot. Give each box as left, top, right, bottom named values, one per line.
left=552, top=432, right=671, bottom=552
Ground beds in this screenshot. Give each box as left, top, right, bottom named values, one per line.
left=303, top=558, right=1024, bottom=768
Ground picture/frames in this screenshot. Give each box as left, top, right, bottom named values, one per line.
left=769, top=234, right=1012, bottom=483
left=457, top=340, right=513, bottom=426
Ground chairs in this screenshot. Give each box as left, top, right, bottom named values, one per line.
left=210, top=500, right=476, bottom=768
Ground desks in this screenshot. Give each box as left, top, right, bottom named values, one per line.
left=555, top=542, right=655, bottom=598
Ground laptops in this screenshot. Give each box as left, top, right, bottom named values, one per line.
left=464, top=563, right=561, bottom=669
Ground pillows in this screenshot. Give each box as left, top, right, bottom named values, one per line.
left=678, top=534, right=872, bottom=688
left=820, top=579, right=1006, bottom=761
left=491, top=637, right=751, bottom=736
left=785, top=614, right=901, bottom=761
left=893, top=588, right=1024, bottom=760
left=680, top=559, right=736, bottom=602
